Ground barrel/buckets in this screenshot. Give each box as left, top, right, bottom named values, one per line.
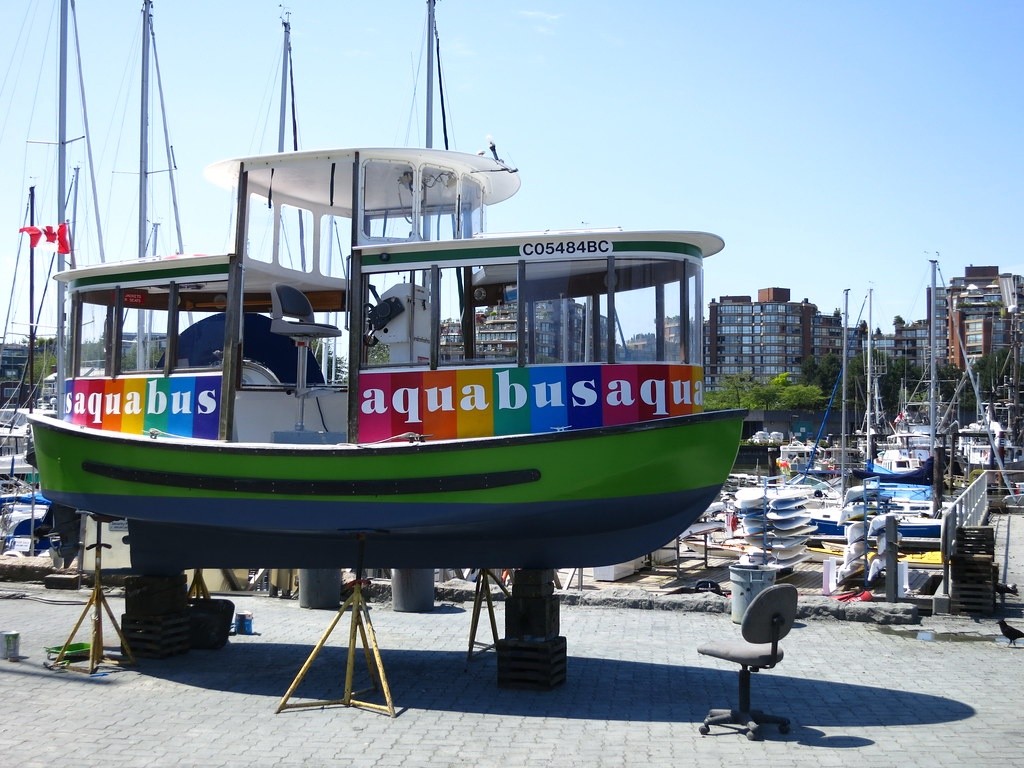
left=0, top=631, right=19, bottom=658
left=299, top=568, right=341, bottom=609
left=235, top=611, right=253, bottom=634
left=729, top=564, right=777, bottom=624
left=391, top=568, right=434, bottom=612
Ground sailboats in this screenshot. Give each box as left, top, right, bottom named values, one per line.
left=0, top=162, right=1024, bottom=584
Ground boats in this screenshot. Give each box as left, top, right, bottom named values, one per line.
left=22, top=0, right=751, bottom=570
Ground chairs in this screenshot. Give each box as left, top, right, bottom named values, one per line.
left=697, top=584, right=799, bottom=742
left=269, top=282, right=348, bottom=445
